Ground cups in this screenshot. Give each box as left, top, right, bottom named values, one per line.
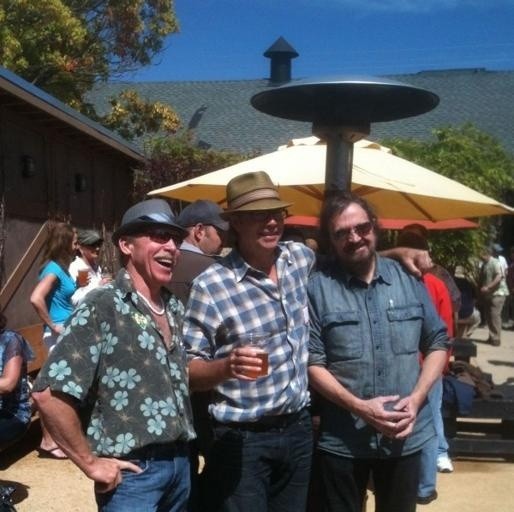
left=76, top=268, right=89, bottom=287
left=100, top=265, right=113, bottom=279
left=236, top=332, right=271, bottom=381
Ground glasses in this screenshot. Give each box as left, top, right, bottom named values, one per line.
left=333, top=221, right=371, bottom=240
left=150, top=228, right=183, bottom=247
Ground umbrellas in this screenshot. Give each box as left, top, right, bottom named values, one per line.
left=281, top=215, right=481, bottom=233
left=144, top=132, right=513, bottom=220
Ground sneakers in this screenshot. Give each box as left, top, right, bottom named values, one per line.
left=437, top=457, right=453, bottom=472
left=416, top=490, right=437, bottom=504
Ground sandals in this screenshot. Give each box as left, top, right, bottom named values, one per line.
left=35, top=446, right=69, bottom=459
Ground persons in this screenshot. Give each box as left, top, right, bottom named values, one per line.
left=180, top=166, right=434, bottom=511
left=28, top=194, right=204, bottom=511
left=172, top=204, right=230, bottom=302
left=0, top=311, right=34, bottom=444
left=397, top=223, right=511, bottom=470
left=31, top=224, right=116, bottom=459
left=306, top=192, right=450, bottom=512
left=392, top=221, right=440, bottom=501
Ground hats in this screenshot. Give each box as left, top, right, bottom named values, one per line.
left=219, top=170, right=294, bottom=221
left=178, top=198, right=230, bottom=231
left=112, top=198, right=190, bottom=247
left=78, top=230, right=103, bottom=245
